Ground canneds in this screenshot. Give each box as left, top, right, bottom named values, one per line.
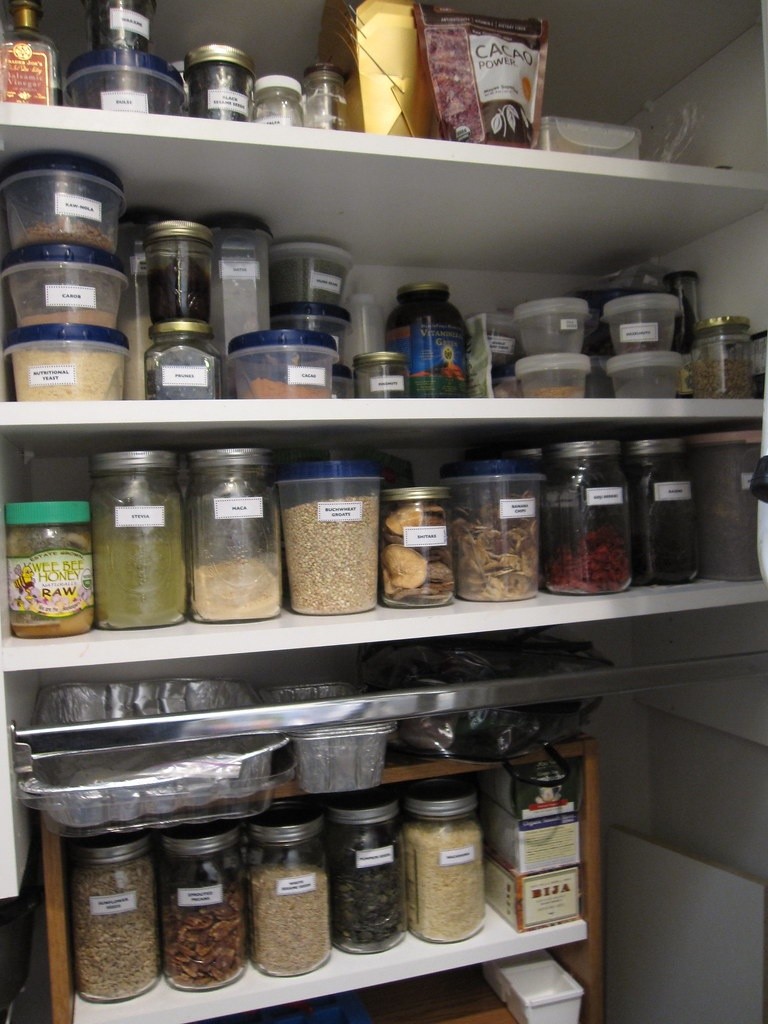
left=71, top=784, right=485, bottom=1005
left=141, top=220, right=757, bottom=400
left=180, top=42, right=349, bottom=131
left=3, top=433, right=698, bottom=640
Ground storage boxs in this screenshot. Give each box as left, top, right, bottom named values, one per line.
left=537, top=114, right=642, bottom=160
left=478, top=762, right=583, bottom=933
left=482, top=950, right=584, bottom=1024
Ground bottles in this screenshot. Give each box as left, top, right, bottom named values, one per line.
left=0, top=0, right=350, bottom=133
left=68, top=782, right=487, bottom=1005
left=3, top=430, right=705, bottom=639
left=0, top=146, right=753, bottom=400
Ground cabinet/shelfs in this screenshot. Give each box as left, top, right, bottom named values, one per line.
left=40, top=737, right=604, bottom=1024
left=0, top=0, right=768, bottom=901
